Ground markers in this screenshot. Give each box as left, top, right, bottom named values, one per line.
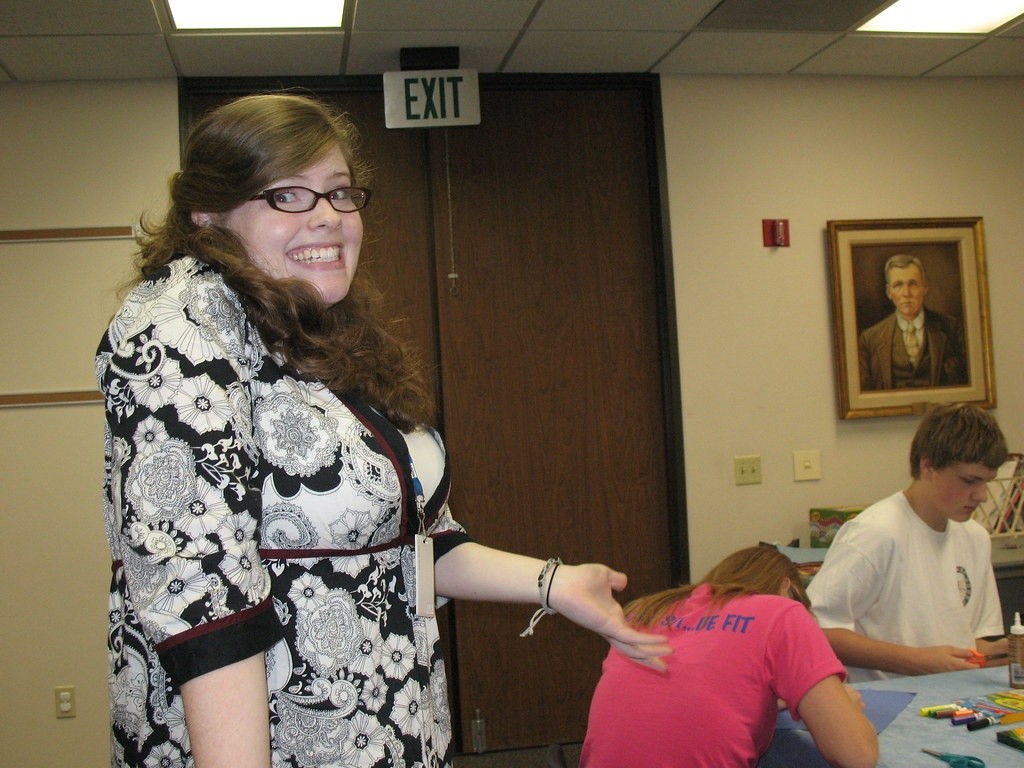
left=919, top=703, right=1000, bottom=733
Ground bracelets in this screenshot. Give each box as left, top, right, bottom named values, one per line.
left=520, top=556, right=563, bottom=637
left=546, top=563, right=560, bottom=608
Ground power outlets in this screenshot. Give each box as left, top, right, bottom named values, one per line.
left=55, top=686, right=75, bottom=719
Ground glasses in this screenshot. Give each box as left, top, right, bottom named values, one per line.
left=248, top=185, right=373, bottom=213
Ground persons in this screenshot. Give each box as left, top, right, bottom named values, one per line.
left=804, top=401, right=1009, bottom=684
left=96, top=94, right=674, bottom=768
left=580, top=546, right=880, bottom=768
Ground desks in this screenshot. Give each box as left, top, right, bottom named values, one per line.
left=988, top=531, right=1024, bottom=634
left=753, top=663, right=1024, bottom=768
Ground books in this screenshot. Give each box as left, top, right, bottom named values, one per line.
left=971, top=460, right=1024, bottom=534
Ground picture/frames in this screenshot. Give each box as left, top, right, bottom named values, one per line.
left=826, top=215, right=997, bottom=422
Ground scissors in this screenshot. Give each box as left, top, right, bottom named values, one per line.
left=965, top=648, right=1009, bottom=668
left=921, top=747, right=986, bottom=768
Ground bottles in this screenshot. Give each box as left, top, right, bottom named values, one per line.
left=1007, top=612, right=1024, bottom=689
left=470, top=709, right=486, bottom=754
